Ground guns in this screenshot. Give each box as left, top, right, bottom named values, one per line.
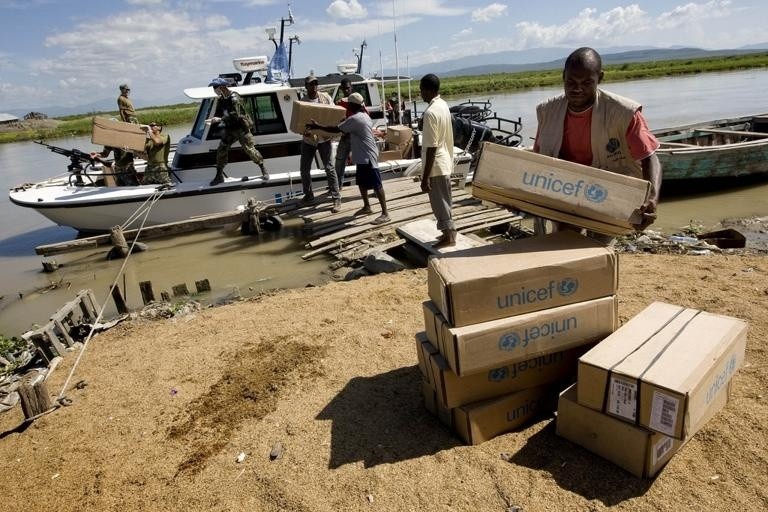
left=33, top=140, right=112, bottom=168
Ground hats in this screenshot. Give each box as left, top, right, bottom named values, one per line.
left=207, top=77, right=232, bottom=89
left=304, top=75, right=318, bottom=89
left=119, top=84, right=131, bottom=94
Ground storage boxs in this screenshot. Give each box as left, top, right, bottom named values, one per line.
left=378, top=125, right=413, bottom=163
left=575, top=302, right=748, bottom=441
left=289, top=100, right=347, bottom=137
left=102, top=166, right=117, bottom=186
left=556, top=376, right=732, bottom=482
left=91, top=116, right=148, bottom=153
left=472, top=141, right=649, bottom=240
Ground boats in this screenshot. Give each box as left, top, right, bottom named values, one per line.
left=7, top=5, right=524, bottom=238
left=648, top=107, right=767, bottom=181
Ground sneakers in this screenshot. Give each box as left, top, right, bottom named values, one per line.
left=296, top=194, right=316, bottom=209
left=370, top=214, right=392, bottom=225
left=430, top=233, right=456, bottom=249
left=332, top=196, right=343, bottom=213
left=353, top=208, right=372, bottom=219
left=340, top=91, right=365, bottom=108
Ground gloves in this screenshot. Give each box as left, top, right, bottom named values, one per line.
left=210, top=117, right=223, bottom=123
left=140, top=124, right=154, bottom=133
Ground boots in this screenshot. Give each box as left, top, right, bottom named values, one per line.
left=209, top=167, right=225, bottom=185
left=259, top=164, right=270, bottom=182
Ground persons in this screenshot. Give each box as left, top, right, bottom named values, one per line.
left=495, top=47, right=663, bottom=248
left=118, top=84, right=139, bottom=124
left=419, top=74, right=457, bottom=249
left=326, top=78, right=370, bottom=196
left=120, top=121, right=176, bottom=185
left=294, top=75, right=342, bottom=213
left=208, top=78, right=269, bottom=186
left=305, top=92, right=389, bottom=225
left=89, top=118, right=140, bottom=186
left=385, top=92, right=405, bottom=125
